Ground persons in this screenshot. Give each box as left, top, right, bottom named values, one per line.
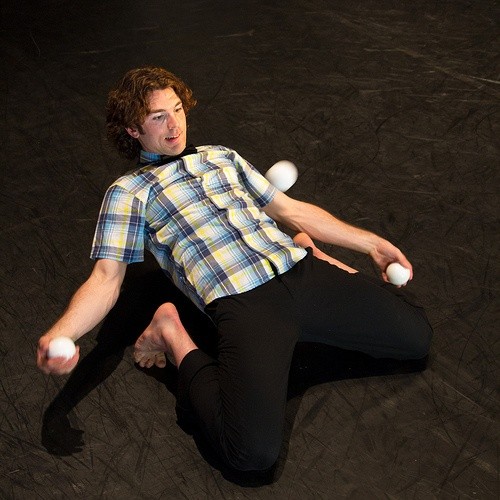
left=36, top=66, right=433, bottom=482
